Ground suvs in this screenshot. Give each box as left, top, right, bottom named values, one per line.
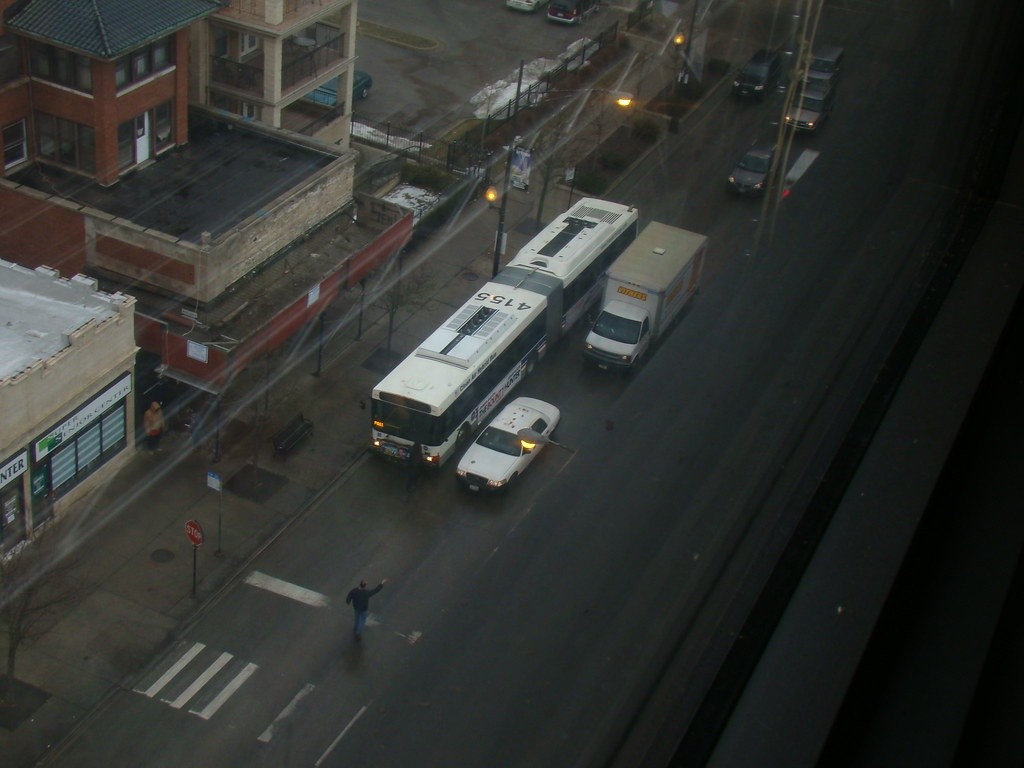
left=785, top=83, right=836, bottom=135
left=733, top=50, right=784, bottom=97
left=548, top=0, right=600, bottom=25
left=804, top=46, right=848, bottom=87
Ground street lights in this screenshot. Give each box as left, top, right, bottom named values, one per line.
left=493, top=93, right=637, bottom=276
left=675, top=27, right=693, bottom=113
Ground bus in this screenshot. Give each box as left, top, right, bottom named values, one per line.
left=358, top=197, right=638, bottom=472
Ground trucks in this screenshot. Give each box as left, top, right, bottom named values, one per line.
left=583, top=221, right=713, bottom=370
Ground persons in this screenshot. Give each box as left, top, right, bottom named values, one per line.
left=145, top=401, right=165, bottom=455
left=347, top=579, right=390, bottom=639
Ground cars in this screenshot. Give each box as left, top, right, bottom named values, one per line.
left=725, top=135, right=779, bottom=200
left=458, top=395, right=562, bottom=503
left=506, top=0, right=546, bottom=12
left=306, top=69, right=373, bottom=106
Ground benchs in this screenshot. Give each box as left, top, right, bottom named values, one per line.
left=270, top=412, right=314, bottom=453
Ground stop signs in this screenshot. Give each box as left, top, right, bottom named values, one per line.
left=183, top=519, right=205, bottom=549
